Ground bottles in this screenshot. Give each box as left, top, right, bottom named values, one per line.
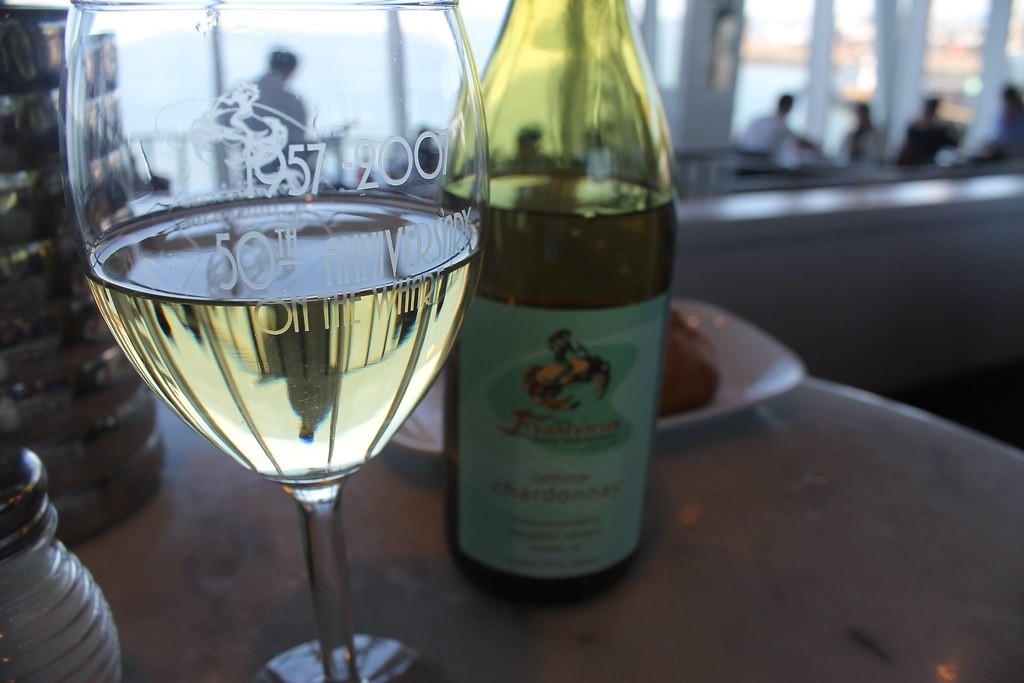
left=0, top=442, right=122, bottom=683
left=442, top=0, right=678, bottom=601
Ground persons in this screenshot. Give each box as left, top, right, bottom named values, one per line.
left=982, top=85, right=1024, bottom=163
left=895, top=98, right=962, bottom=167
left=215, top=51, right=308, bottom=183
left=840, top=103, right=885, bottom=164
left=407, top=127, right=614, bottom=179
left=738, top=95, right=816, bottom=167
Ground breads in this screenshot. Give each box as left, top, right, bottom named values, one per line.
left=657, top=310, right=722, bottom=416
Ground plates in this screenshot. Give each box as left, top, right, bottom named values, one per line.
left=389, top=299, right=806, bottom=458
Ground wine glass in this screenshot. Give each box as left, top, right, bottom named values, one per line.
left=65, top=0, right=489, bottom=683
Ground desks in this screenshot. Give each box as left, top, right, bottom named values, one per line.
left=66, top=381, right=1024, bottom=683
